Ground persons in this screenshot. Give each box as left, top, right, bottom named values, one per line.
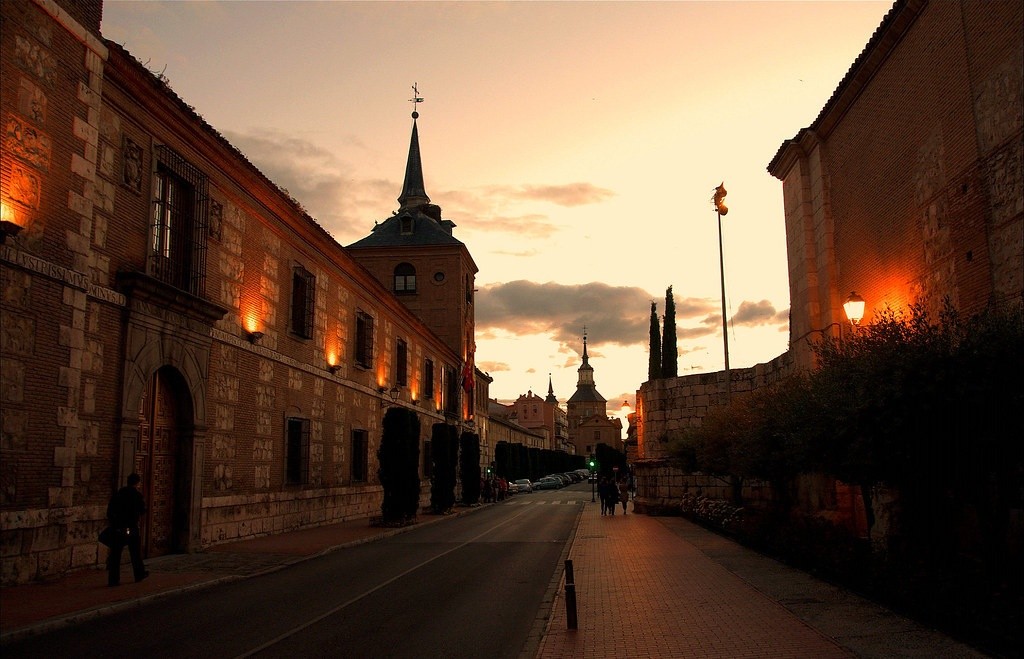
left=104, top=473, right=151, bottom=587
left=607, top=480, right=619, bottom=515
left=597, top=479, right=608, bottom=516
left=619, top=479, right=629, bottom=515
left=484, top=476, right=492, bottom=503
left=500, top=474, right=508, bottom=499
left=492, top=476, right=500, bottom=502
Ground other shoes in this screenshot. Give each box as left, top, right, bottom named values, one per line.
left=109, top=582, right=118, bottom=586
left=135, top=571, right=149, bottom=582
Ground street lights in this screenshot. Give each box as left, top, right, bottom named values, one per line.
left=710, top=177, right=733, bottom=372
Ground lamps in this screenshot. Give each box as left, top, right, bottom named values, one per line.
left=248, top=331, right=264, bottom=343
left=413, top=399, right=421, bottom=405
left=379, top=386, right=388, bottom=393
left=464, top=419, right=475, bottom=431
left=330, top=365, right=341, bottom=372
left=842, top=291, right=879, bottom=329
left=0, top=220, right=26, bottom=237
left=436, top=410, right=446, bottom=413
left=380, top=384, right=400, bottom=409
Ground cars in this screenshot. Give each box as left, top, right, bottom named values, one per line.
left=588, top=472, right=597, bottom=484
left=514, top=479, right=533, bottom=493
left=532, top=477, right=563, bottom=490
left=549, top=468, right=590, bottom=487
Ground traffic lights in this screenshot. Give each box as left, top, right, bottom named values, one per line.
left=589, top=461, right=595, bottom=467
left=487, top=467, right=491, bottom=474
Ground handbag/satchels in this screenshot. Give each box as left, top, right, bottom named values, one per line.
left=98, top=527, right=116, bottom=548
